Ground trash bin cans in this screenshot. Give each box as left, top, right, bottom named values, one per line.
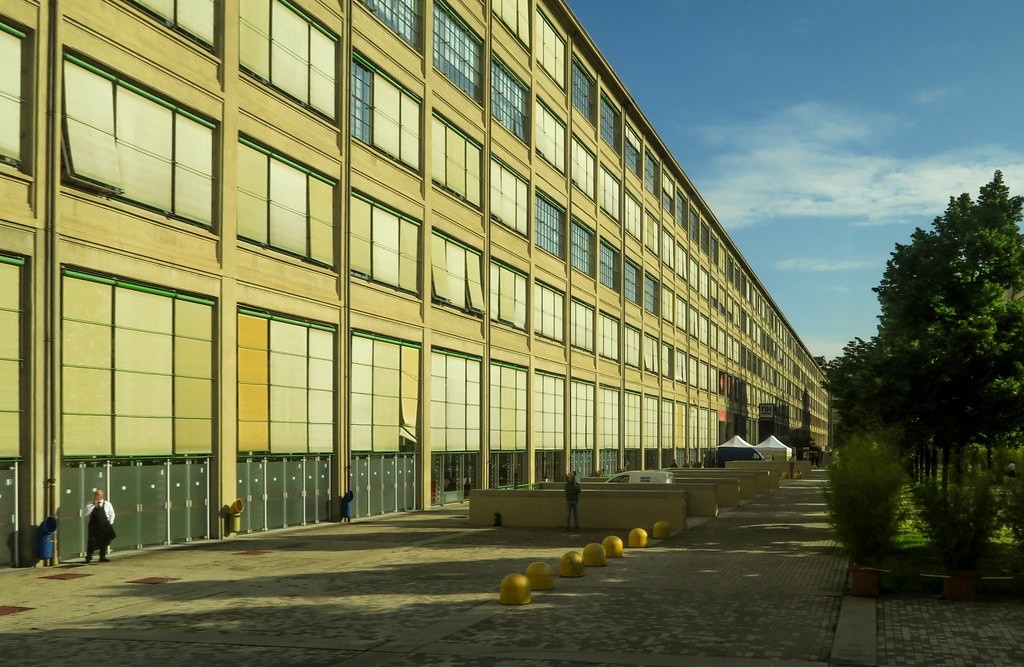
left=342, top=490, right=354, bottom=517
left=38, top=516, right=58, bottom=560
left=228, top=498, right=245, bottom=532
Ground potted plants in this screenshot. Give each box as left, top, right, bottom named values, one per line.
left=910, top=464, right=999, bottom=602
left=824, top=433, right=903, bottom=597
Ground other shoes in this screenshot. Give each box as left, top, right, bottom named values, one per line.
left=99, top=558, right=110, bottom=562
left=563, top=528, right=569, bottom=532
left=86, top=556, right=91, bottom=563
left=575, top=527, right=583, bottom=532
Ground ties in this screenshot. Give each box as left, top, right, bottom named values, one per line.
left=98, top=502, right=100, bottom=507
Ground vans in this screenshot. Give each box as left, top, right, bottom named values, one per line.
left=604, top=470, right=674, bottom=485
left=444, top=468, right=471, bottom=488
left=717, top=447, right=764, bottom=468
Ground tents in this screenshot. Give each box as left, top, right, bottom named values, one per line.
left=754, top=435, right=792, bottom=461
left=715, top=436, right=754, bottom=468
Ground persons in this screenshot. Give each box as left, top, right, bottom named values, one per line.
left=84, top=490, right=117, bottom=564
left=809, top=439, right=821, bottom=468
left=564, top=474, right=583, bottom=532
left=1008, top=461, right=1015, bottom=477
left=670, top=459, right=678, bottom=467
